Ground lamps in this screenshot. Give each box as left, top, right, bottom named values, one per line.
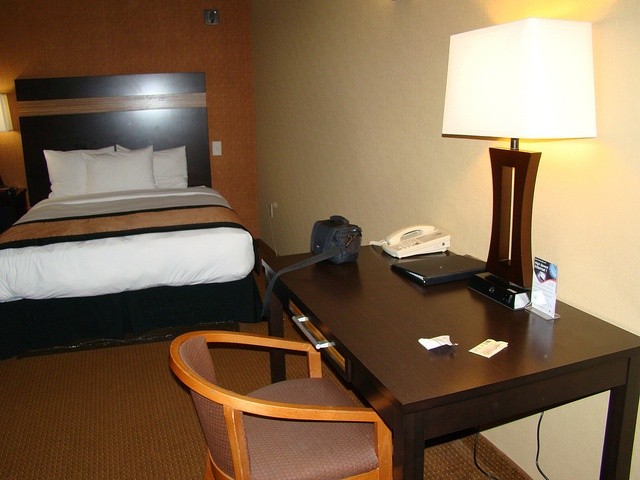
left=442, top=18, right=598, bottom=288
left=0, top=93, right=14, bottom=133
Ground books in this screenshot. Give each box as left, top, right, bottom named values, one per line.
left=389, top=254, right=487, bottom=287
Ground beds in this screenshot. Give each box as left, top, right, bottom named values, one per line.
left=1, top=72, right=265, bottom=359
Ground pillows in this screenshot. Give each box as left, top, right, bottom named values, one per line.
left=42, top=146, right=115, bottom=199
left=116, top=144, right=189, bottom=190
left=79, top=145, right=157, bottom=191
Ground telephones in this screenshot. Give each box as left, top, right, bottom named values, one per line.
left=368, top=225, right=452, bottom=259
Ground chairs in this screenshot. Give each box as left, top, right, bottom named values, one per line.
left=168, top=329, right=393, bottom=479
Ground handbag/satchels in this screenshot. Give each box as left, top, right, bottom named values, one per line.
left=310, top=213, right=362, bottom=263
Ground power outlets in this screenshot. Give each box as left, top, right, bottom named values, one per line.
left=204, top=8, right=219, bottom=26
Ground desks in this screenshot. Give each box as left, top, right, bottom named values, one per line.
left=261, top=247, right=640, bottom=479
